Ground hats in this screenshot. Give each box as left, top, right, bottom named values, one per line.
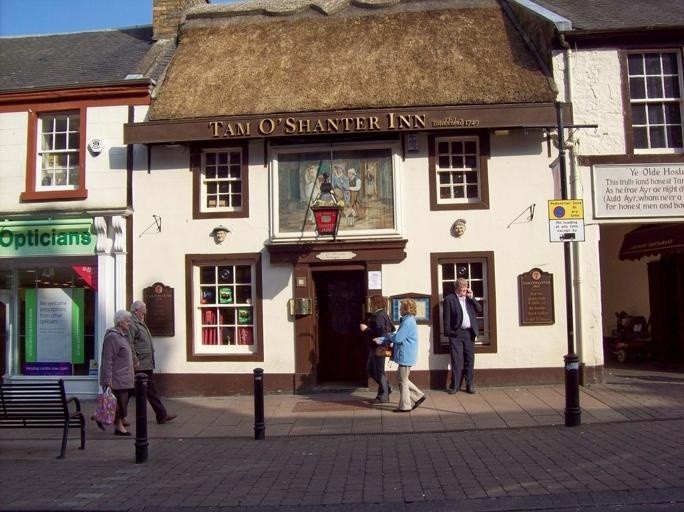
left=334, top=162, right=347, bottom=171
left=348, top=168, right=357, bottom=175
left=213, top=225, right=230, bottom=232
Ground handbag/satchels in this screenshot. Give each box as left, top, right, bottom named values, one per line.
left=94, top=388, right=117, bottom=424
left=375, top=335, right=392, bottom=357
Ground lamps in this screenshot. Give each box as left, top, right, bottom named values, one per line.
left=310, top=173, right=344, bottom=242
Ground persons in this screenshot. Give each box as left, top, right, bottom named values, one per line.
left=357, top=293, right=395, bottom=404
left=212, top=224, right=229, bottom=243
left=113, top=299, right=179, bottom=427
left=344, top=168, right=365, bottom=221
left=303, top=165, right=321, bottom=224
left=452, top=219, right=467, bottom=235
left=332, top=162, right=351, bottom=206
left=442, top=278, right=483, bottom=396
left=371, top=298, right=426, bottom=415
left=87, top=306, right=135, bottom=436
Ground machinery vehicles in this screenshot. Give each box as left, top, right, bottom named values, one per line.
left=606, top=313, right=655, bottom=365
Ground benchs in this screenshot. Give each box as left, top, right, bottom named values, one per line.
left=0, top=379, right=86, bottom=458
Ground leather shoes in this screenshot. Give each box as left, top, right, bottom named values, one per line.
left=158, top=415, right=178, bottom=425
left=370, top=388, right=392, bottom=404
left=466, top=388, right=475, bottom=394
left=392, top=408, right=412, bottom=412
left=91, top=415, right=106, bottom=431
left=448, top=388, right=459, bottom=394
left=113, top=418, right=132, bottom=436
left=412, top=396, right=426, bottom=410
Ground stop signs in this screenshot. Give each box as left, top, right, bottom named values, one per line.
left=548, top=200, right=586, bottom=243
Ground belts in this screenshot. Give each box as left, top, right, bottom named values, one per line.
left=460, top=328, right=471, bottom=331
